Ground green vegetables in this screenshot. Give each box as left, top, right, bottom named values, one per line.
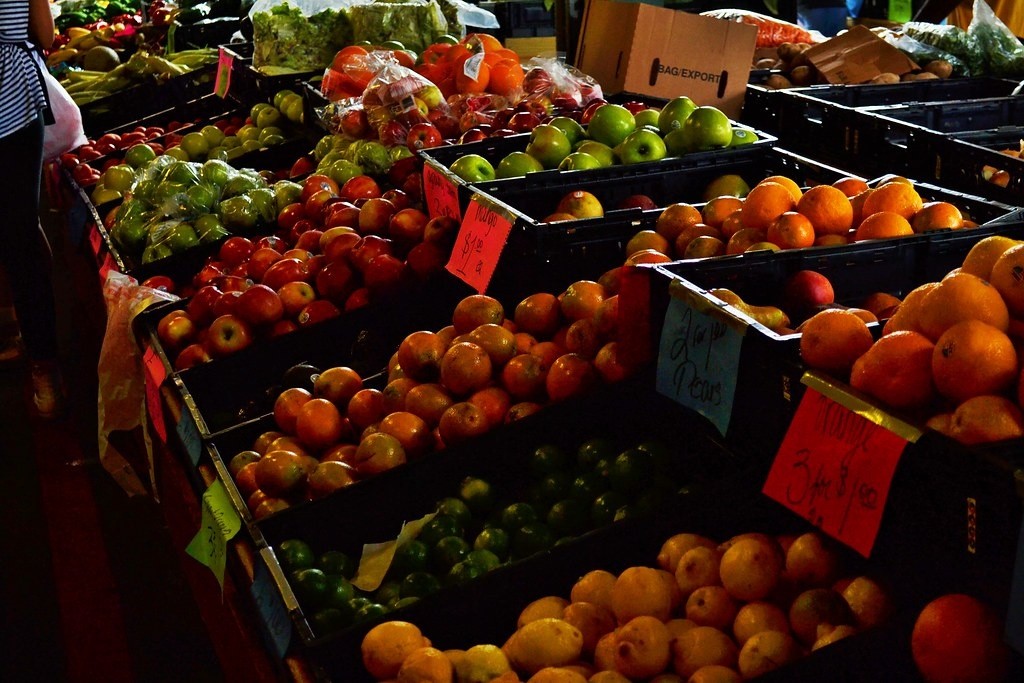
left=251, top=0, right=463, bottom=79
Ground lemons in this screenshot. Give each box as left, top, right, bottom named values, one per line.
left=361, top=530, right=884, bottom=682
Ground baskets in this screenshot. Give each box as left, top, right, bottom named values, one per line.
left=33, top=2, right=1022, bottom=682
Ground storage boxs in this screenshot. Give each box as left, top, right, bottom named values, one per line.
left=31, top=0, right=1024, bottom=683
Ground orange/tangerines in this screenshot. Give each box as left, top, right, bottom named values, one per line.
left=229, top=174, right=1024, bottom=639
left=323, top=33, right=525, bottom=100
left=907, top=593, right=1000, bottom=678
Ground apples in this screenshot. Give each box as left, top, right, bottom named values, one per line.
left=335, top=67, right=760, bottom=183
left=92, top=91, right=461, bottom=370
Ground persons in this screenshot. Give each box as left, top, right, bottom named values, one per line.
left=0, top=1, right=59, bottom=419
